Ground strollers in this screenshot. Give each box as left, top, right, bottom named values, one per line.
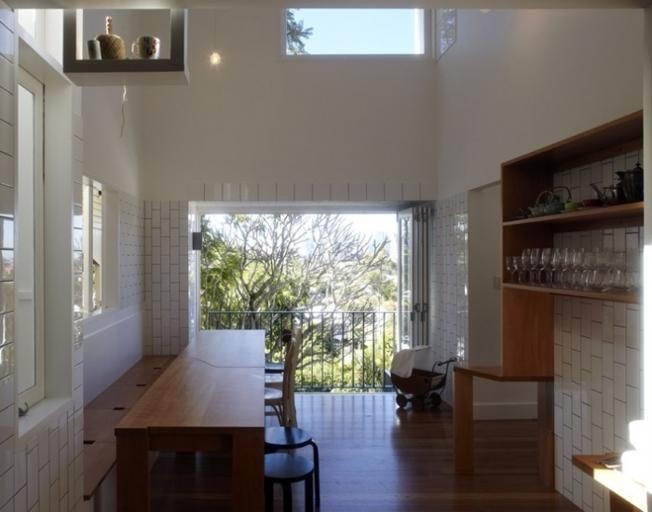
left=390, top=346, right=456, bottom=410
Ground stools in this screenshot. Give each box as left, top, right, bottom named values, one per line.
left=262, top=452, right=313, bottom=511
left=265, top=424, right=319, bottom=510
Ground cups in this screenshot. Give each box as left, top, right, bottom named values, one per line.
left=131, top=35, right=162, bottom=59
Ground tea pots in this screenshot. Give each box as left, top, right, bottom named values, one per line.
left=529, top=197, right=563, bottom=216
left=615, top=168, right=643, bottom=204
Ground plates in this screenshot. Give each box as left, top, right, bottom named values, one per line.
left=559, top=206, right=599, bottom=213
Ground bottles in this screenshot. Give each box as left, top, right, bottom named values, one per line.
left=85, top=40, right=105, bottom=60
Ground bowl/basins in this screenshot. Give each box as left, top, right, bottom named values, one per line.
left=564, top=199, right=604, bottom=206
left=602, top=184, right=623, bottom=202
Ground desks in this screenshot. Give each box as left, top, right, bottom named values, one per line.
left=114, top=329, right=267, bottom=509
left=571, top=453, right=652, bottom=512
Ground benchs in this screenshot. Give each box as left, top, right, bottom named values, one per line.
left=82, top=352, right=178, bottom=512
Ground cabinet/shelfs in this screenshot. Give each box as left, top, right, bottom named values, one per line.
left=449, top=106, right=644, bottom=480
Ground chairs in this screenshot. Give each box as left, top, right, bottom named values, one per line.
left=262, top=317, right=302, bottom=428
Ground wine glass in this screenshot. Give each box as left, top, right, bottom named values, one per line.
left=506, top=246, right=642, bottom=294
